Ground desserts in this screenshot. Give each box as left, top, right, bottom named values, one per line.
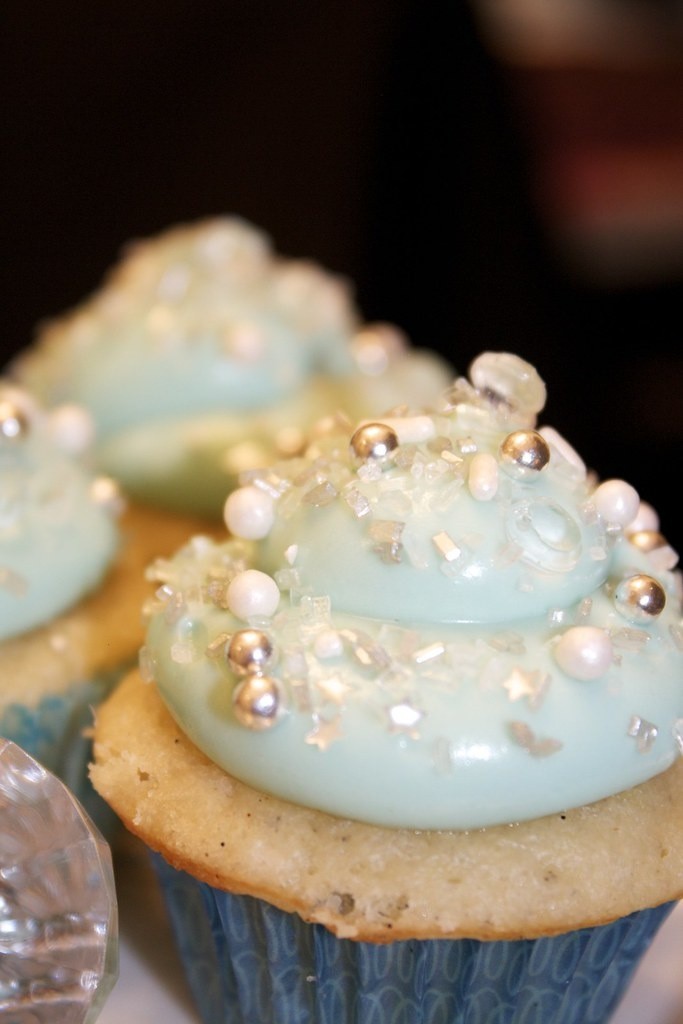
left=0, top=219, right=683, bottom=1024
